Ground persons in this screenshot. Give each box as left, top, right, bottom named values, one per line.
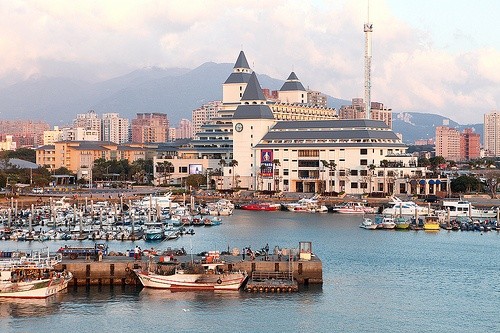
left=242, top=247, right=255, bottom=261
left=265, top=243, right=269, bottom=255
left=86, top=251, right=91, bottom=260
left=98, top=250, right=103, bottom=262
left=134, top=246, right=141, bottom=260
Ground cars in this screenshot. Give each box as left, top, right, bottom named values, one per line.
left=445, top=193, right=464, bottom=198
left=424, top=195, right=441, bottom=203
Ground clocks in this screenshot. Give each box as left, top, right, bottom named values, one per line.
left=235, top=123, right=243, bottom=132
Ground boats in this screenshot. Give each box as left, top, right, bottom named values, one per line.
left=131, top=243, right=249, bottom=291
left=238, top=200, right=276, bottom=212
left=333, top=199, right=500, bottom=231
left=0, top=247, right=73, bottom=299
left=280, top=198, right=329, bottom=214
left=260, top=202, right=282, bottom=210
left=0, top=193, right=235, bottom=241
